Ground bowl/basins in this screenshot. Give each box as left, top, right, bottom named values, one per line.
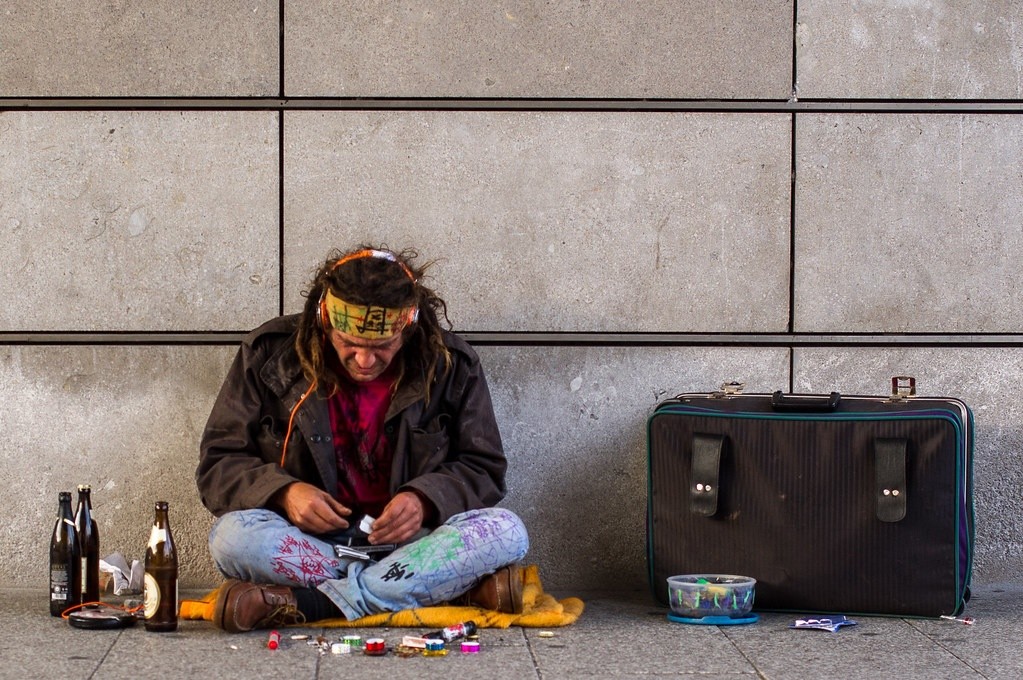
left=665, top=574, right=759, bottom=625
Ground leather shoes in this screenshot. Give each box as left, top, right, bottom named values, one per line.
left=213, top=577, right=300, bottom=634
left=449, top=562, right=523, bottom=615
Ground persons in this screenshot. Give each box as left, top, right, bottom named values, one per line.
left=191, top=245, right=533, bottom=635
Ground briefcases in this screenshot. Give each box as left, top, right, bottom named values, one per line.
left=644, top=375, right=973, bottom=615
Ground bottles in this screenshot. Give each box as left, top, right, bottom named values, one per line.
left=50, top=491, right=82, bottom=617
left=73, top=485, right=100, bottom=609
left=143, top=501, right=179, bottom=632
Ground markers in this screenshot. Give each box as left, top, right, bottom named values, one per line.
left=268, top=630, right=281, bottom=651
